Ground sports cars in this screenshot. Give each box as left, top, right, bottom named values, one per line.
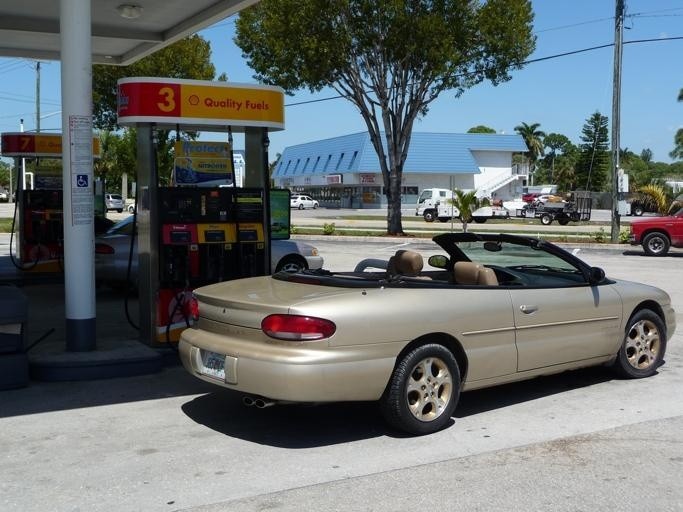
left=178, top=232, right=676, bottom=435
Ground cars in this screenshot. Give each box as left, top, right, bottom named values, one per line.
left=94, top=214, right=324, bottom=292
left=105, top=194, right=136, bottom=214
left=630, top=207, right=683, bottom=256
left=291, top=194, right=319, bottom=210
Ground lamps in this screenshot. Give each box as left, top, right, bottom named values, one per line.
left=116, top=5, right=144, bottom=18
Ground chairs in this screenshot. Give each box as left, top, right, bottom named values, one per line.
left=387, top=250, right=432, bottom=281
left=454, top=261, right=499, bottom=286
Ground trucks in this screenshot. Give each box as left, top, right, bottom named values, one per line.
left=415, top=188, right=509, bottom=223
